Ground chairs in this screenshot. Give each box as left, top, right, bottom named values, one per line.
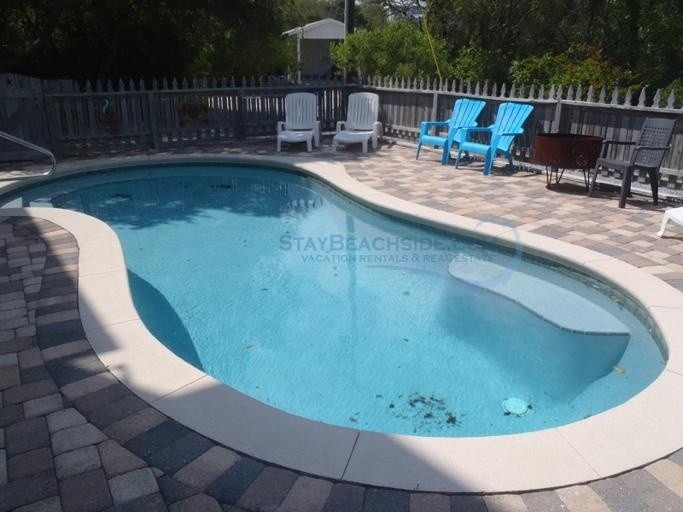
left=331, top=92, right=383, bottom=152
left=456, top=102, right=534, bottom=175
left=657, top=205, right=683, bottom=236
left=588, top=117, right=677, bottom=209
left=416, top=98, right=487, bottom=166
left=277, top=92, right=319, bottom=153
